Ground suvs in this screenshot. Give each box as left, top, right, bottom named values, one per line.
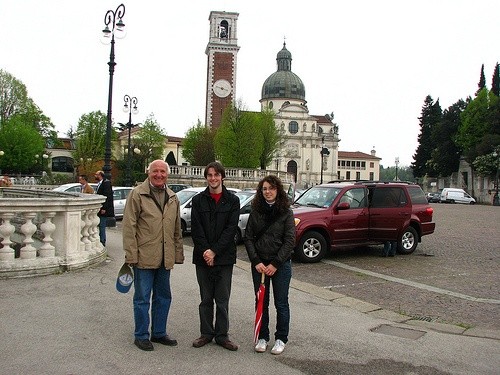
left=291, top=180, right=435, bottom=263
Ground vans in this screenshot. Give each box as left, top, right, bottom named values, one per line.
left=440, top=187, right=476, bottom=205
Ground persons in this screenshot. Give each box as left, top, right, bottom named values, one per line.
left=242, top=175, right=295, bottom=355
left=121, top=158, right=186, bottom=351
left=78, top=174, right=94, bottom=195
left=382, top=187, right=405, bottom=257
left=91, top=170, right=114, bottom=249
left=0, top=174, right=13, bottom=187
left=187, top=161, right=241, bottom=351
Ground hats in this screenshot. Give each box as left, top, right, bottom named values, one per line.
left=116, top=262, right=134, bottom=294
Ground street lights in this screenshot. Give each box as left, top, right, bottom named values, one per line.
left=99, top=4, right=128, bottom=228
left=34, top=152, right=48, bottom=174
left=125, top=143, right=140, bottom=169
left=123, top=95, right=139, bottom=187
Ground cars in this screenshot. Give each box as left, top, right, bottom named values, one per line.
left=50, top=182, right=353, bottom=245
left=423, top=191, right=441, bottom=203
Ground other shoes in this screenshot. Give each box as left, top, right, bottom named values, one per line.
left=216, top=338, right=238, bottom=351
left=134, top=338, right=154, bottom=351
left=150, top=334, right=178, bottom=346
left=271, top=339, right=285, bottom=355
left=255, top=339, right=269, bottom=352
left=193, top=336, right=213, bottom=347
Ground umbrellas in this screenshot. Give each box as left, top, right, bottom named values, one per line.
left=253, top=271, right=266, bottom=355
